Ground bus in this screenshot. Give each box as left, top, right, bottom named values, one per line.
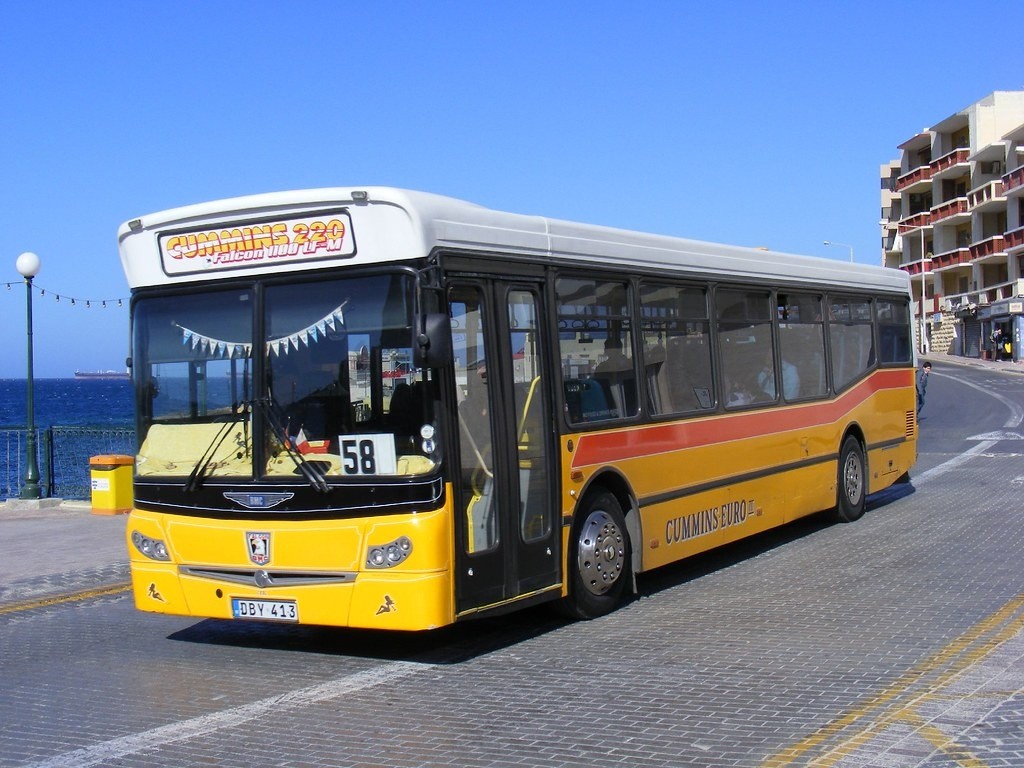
left=114, top=184, right=918, bottom=631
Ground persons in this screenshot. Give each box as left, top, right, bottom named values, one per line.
left=991, top=328, right=999, bottom=362
left=594, top=338, right=629, bottom=374
left=915, top=362, right=932, bottom=422
left=758, top=344, right=800, bottom=400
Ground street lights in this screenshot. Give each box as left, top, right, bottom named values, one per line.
left=15, top=252, right=41, bottom=500
left=823, top=241, right=854, bottom=261
left=879, top=222, right=924, bottom=354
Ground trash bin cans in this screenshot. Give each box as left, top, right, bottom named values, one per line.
left=87, top=450, right=135, bottom=516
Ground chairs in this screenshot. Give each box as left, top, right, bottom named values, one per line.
left=564, top=378, right=611, bottom=421
left=792, top=327, right=902, bottom=397
